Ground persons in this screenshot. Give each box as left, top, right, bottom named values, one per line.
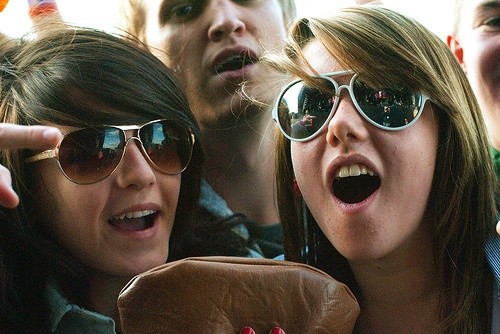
left=130, top=0, right=500, bottom=334
left=0, top=28, right=265, bottom=334
